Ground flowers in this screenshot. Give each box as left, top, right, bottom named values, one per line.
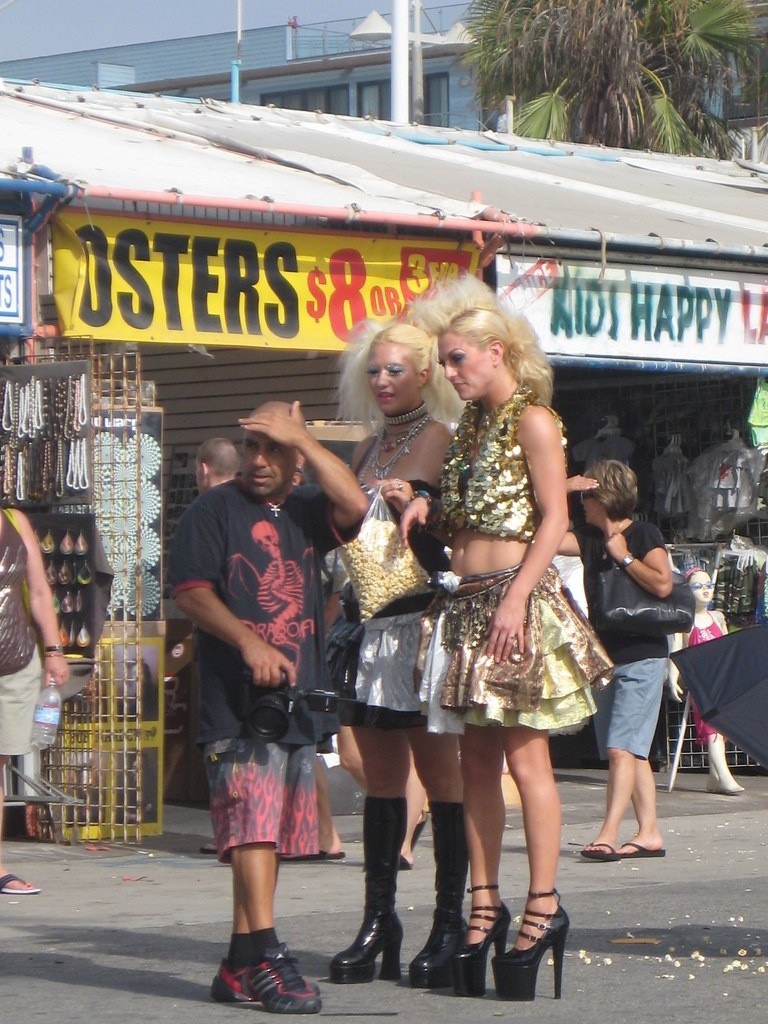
left=722, top=538, right=754, bottom=557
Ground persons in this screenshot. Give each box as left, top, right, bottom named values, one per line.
left=0, top=506, right=71, bottom=896
left=166, top=265, right=617, bottom=1016
left=555, top=457, right=675, bottom=863
left=667, top=566, right=746, bottom=795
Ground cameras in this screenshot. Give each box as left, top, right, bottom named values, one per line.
left=241, top=668, right=339, bottom=745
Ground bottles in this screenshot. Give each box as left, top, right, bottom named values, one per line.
left=30, top=677, right=61, bottom=750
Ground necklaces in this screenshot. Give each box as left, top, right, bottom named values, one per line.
left=234, top=471, right=293, bottom=518
left=0, top=372, right=90, bottom=504
left=600, top=519, right=631, bottom=560
left=359, top=400, right=433, bottom=496
left=469, top=381, right=520, bottom=453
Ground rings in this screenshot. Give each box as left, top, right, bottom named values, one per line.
left=287, top=661, right=294, bottom=672
left=394, top=477, right=404, bottom=489
left=507, top=636, right=514, bottom=640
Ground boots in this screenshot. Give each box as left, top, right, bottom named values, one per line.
left=409, top=801, right=469, bottom=988
left=330, top=797, right=404, bottom=982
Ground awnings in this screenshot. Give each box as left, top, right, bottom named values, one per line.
left=51, top=185, right=508, bottom=352
left=0, top=178, right=68, bottom=337
left=494, top=217, right=768, bottom=377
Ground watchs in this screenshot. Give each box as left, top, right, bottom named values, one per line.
left=620, top=553, right=640, bottom=571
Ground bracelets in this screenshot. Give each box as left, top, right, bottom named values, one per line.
left=42, top=644, right=66, bottom=659
left=408, top=489, right=443, bottom=534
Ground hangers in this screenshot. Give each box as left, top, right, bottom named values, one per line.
left=663, top=434, right=682, bottom=457
left=720, top=428, right=743, bottom=453
left=594, top=415, right=621, bottom=439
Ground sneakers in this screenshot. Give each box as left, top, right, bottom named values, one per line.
left=209, top=958, right=260, bottom=1001
left=241, top=941, right=322, bottom=1014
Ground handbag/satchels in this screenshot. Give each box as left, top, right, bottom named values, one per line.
left=588, top=519, right=697, bottom=635
left=325, top=621, right=364, bottom=693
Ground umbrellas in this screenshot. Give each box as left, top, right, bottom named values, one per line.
left=669, top=625, right=768, bottom=773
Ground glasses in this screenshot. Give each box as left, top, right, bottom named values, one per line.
left=580, top=492, right=595, bottom=500
left=690, top=582, right=717, bottom=590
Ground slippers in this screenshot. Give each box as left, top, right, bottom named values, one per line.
left=411, top=810, right=428, bottom=851
left=0, top=874, right=41, bottom=895
left=619, top=843, right=666, bottom=857
left=285, top=849, right=345, bottom=859
left=364, top=854, right=413, bottom=871
left=580, top=843, right=621, bottom=861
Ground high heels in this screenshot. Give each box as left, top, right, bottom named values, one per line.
left=492, top=905, right=570, bottom=1001
left=453, top=903, right=511, bottom=996
